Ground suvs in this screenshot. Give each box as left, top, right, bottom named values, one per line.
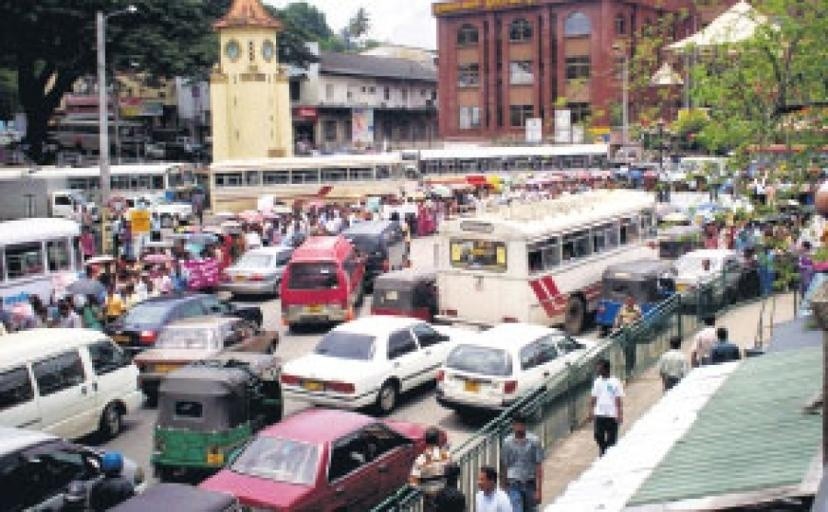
left=436, top=322, right=601, bottom=422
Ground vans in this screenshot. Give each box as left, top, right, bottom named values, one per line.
left=0, top=324, right=148, bottom=445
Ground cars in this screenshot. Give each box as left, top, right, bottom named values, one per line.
left=145, top=137, right=203, bottom=158
left=278, top=313, right=481, bottom=417
left=0, top=425, right=140, bottom=512
left=672, top=247, right=757, bottom=309
left=192, top=406, right=448, bottom=512
left=659, top=213, right=698, bottom=259
left=102, top=292, right=264, bottom=358
left=135, top=313, right=279, bottom=407
left=93, top=478, right=239, bottom=512
left=632, top=137, right=826, bottom=203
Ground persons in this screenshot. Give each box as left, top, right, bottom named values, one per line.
left=1, top=136, right=827, bottom=511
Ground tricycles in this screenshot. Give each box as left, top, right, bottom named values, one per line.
left=594, top=259, right=680, bottom=344
left=147, top=348, right=283, bottom=484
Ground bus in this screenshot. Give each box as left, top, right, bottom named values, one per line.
left=435, top=188, right=658, bottom=336
left=0, top=214, right=86, bottom=315
left=47, top=119, right=146, bottom=156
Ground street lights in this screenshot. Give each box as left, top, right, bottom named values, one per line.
left=654, top=119, right=664, bottom=171
left=93, top=5, right=139, bottom=255
left=609, top=39, right=628, bottom=146
left=112, top=52, right=144, bottom=164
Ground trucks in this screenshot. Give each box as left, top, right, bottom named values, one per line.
left=0, top=169, right=87, bottom=219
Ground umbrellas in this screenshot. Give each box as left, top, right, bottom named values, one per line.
left=651, top=62, right=684, bottom=124
left=661, top=0, right=792, bottom=55
